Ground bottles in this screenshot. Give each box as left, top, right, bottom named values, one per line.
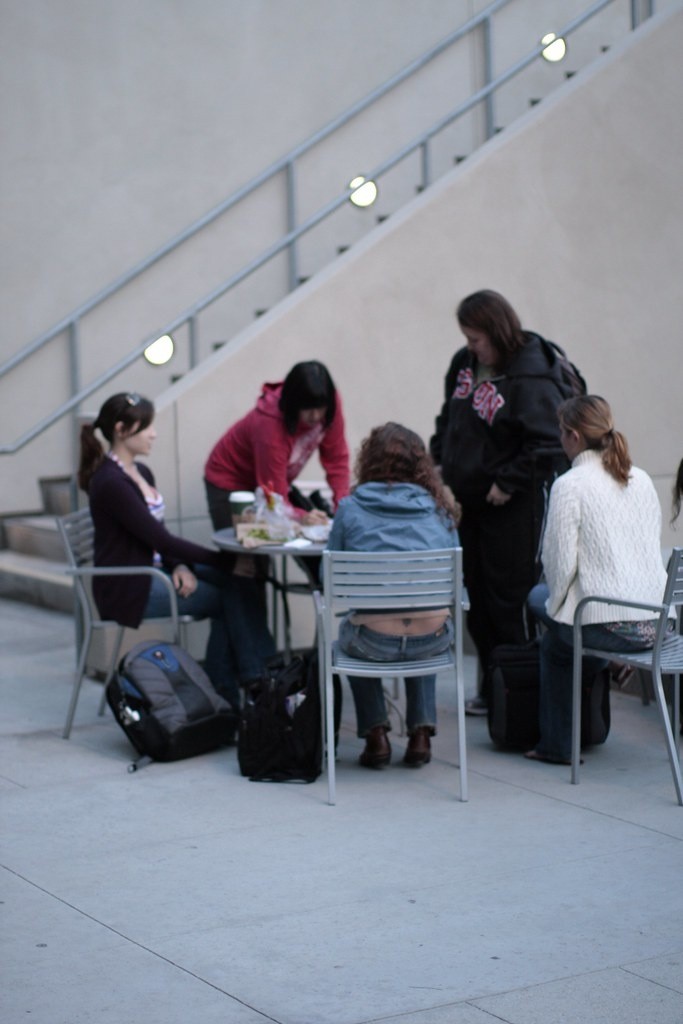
left=116, top=706, right=139, bottom=724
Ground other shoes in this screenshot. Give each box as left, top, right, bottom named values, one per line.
left=525, top=740, right=585, bottom=764
left=465, top=692, right=487, bottom=716
left=403, top=734, right=431, bottom=766
left=359, top=732, right=391, bottom=766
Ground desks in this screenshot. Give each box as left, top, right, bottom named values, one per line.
left=210, top=527, right=328, bottom=665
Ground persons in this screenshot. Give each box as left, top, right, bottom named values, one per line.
left=73, top=390, right=289, bottom=748
left=318, top=419, right=466, bottom=770
left=427, top=288, right=590, bottom=718
left=200, top=359, right=353, bottom=630
left=524, top=394, right=679, bottom=766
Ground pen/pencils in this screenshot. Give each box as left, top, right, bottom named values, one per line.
left=304, top=497, right=317, bottom=511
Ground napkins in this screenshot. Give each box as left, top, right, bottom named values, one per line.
left=283, top=538, right=313, bottom=549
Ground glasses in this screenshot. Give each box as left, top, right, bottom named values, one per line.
left=111, top=392, right=140, bottom=426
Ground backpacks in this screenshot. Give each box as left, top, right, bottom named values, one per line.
left=106, top=639, right=236, bottom=771
left=237, top=653, right=342, bottom=782
left=547, top=340, right=587, bottom=400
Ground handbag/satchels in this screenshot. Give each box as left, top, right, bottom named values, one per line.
left=483, top=639, right=611, bottom=752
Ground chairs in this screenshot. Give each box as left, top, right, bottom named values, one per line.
left=56, top=506, right=192, bottom=739
left=572, top=546, right=683, bottom=806
left=303, top=547, right=468, bottom=805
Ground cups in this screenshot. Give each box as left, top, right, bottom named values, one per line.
left=230, top=492, right=257, bottom=537
